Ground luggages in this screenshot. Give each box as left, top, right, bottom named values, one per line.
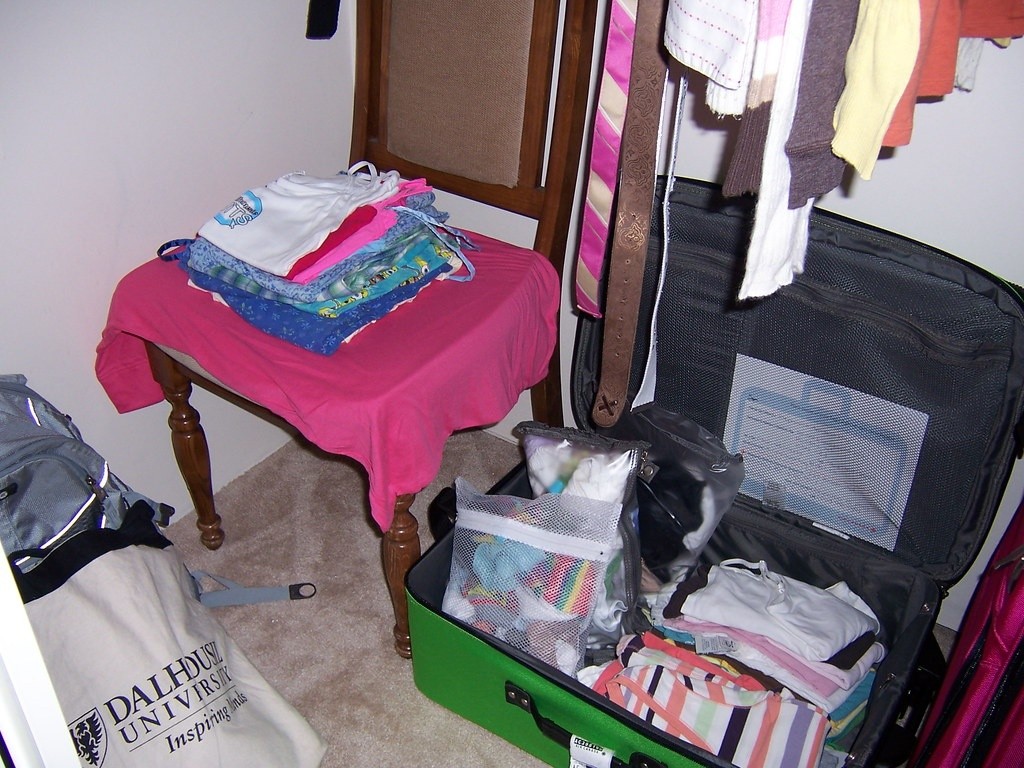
left=402, top=172, right=1024, bottom=768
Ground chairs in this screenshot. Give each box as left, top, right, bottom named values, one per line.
left=120, top=1, right=609, bottom=660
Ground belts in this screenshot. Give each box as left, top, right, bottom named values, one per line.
left=592, top=0, right=670, bottom=428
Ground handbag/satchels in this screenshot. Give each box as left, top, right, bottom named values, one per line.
left=0, top=500, right=328, bottom=768
left=0, top=374, right=317, bottom=606
